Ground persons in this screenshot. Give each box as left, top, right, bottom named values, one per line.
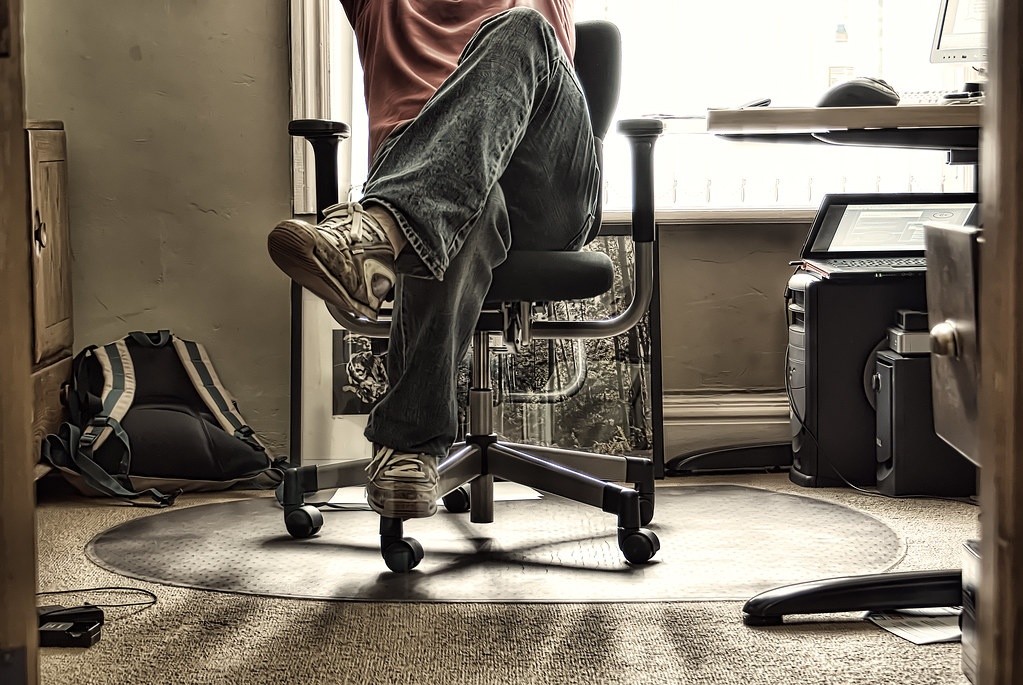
left=267, top=0, right=600, bottom=519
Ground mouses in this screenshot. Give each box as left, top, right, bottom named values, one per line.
left=818, top=77, right=902, bottom=107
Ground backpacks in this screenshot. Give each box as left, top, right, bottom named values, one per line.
left=42, top=332, right=286, bottom=508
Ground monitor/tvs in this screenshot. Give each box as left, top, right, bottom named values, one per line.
left=930, top=0, right=989, bottom=63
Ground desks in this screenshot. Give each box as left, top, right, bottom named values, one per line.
left=706, top=104, right=985, bottom=629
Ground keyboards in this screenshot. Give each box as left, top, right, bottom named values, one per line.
left=943, top=91, right=984, bottom=104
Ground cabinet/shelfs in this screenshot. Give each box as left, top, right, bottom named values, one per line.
left=24, top=119, right=76, bottom=484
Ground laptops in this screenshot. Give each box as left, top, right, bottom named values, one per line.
left=800, top=192, right=980, bottom=279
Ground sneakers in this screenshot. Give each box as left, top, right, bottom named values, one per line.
left=269, top=183, right=396, bottom=324
left=365, top=444, right=439, bottom=519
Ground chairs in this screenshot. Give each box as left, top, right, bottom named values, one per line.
left=283, top=19, right=664, bottom=576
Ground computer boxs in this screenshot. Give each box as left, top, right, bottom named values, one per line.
left=785, top=273, right=927, bottom=488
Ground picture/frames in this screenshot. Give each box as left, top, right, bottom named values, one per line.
left=453, top=223, right=665, bottom=481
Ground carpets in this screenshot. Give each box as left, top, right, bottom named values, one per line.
left=83, top=483, right=908, bottom=606
